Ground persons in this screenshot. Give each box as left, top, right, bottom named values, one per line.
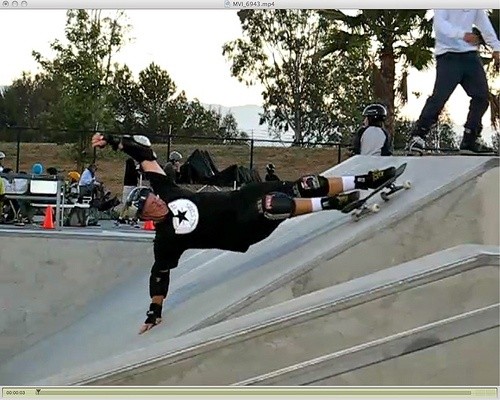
left=90, top=130, right=397, bottom=335
left=0, top=152, right=59, bottom=224
left=264, top=162, right=281, bottom=182
left=163, top=150, right=183, bottom=176
left=64, top=163, right=101, bottom=227
left=351, top=104, right=394, bottom=156
left=118, top=156, right=146, bottom=222
left=401, top=8, right=499, bottom=156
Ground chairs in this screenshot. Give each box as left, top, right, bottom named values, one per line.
left=367, top=167, right=396, bottom=189
left=408, top=136, right=426, bottom=154
left=460, top=139, right=495, bottom=155
left=335, top=191, right=359, bottom=210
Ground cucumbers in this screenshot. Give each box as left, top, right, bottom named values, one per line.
left=342, top=162, right=412, bottom=221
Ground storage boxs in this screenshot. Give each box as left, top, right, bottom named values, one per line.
left=65, top=180, right=94, bottom=227
left=1, top=173, right=64, bottom=226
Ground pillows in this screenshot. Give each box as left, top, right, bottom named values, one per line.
left=362, top=103, right=387, bottom=123
left=0, top=152, right=5, bottom=158
left=168, top=150, right=183, bottom=161
left=266, top=161, right=276, bottom=172
left=68, top=171, right=81, bottom=182
left=125, top=186, right=154, bottom=217
left=33, top=163, right=43, bottom=174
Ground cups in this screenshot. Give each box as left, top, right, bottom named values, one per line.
left=148, top=194, right=161, bottom=210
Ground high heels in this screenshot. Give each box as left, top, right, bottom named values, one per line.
left=144, top=219, right=155, bottom=228
left=45, top=206, right=54, bottom=227
